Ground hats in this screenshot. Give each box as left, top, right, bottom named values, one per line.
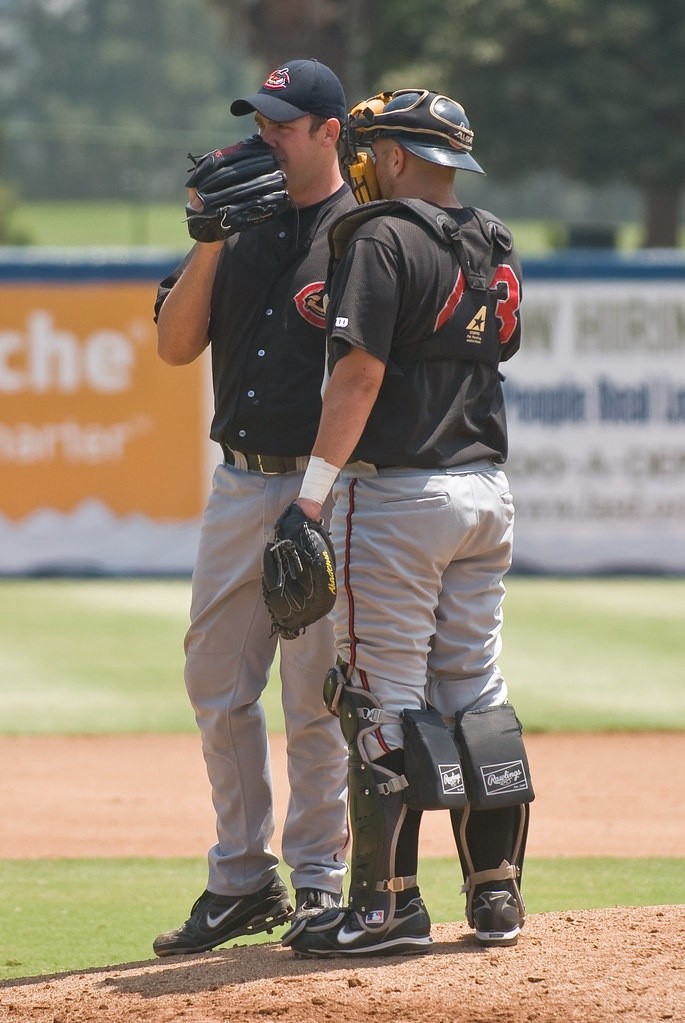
left=229, top=58, right=347, bottom=132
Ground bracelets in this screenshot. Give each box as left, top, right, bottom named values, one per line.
left=297, top=455, right=341, bottom=506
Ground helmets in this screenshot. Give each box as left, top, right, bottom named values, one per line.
left=373, top=92, right=487, bottom=176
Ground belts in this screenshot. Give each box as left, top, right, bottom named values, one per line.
left=219, top=443, right=296, bottom=475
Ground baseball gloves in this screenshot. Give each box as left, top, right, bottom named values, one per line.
left=256, top=503, right=338, bottom=642
left=181, top=130, right=295, bottom=243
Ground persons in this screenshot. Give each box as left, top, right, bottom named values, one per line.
left=152, top=59, right=360, bottom=957
left=261, top=89, right=530, bottom=958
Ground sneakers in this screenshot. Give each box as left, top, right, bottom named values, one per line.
left=289, top=886, right=344, bottom=927
left=152, top=870, right=294, bottom=956
left=471, top=890, right=524, bottom=947
left=279, top=897, right=434, bottom=956
